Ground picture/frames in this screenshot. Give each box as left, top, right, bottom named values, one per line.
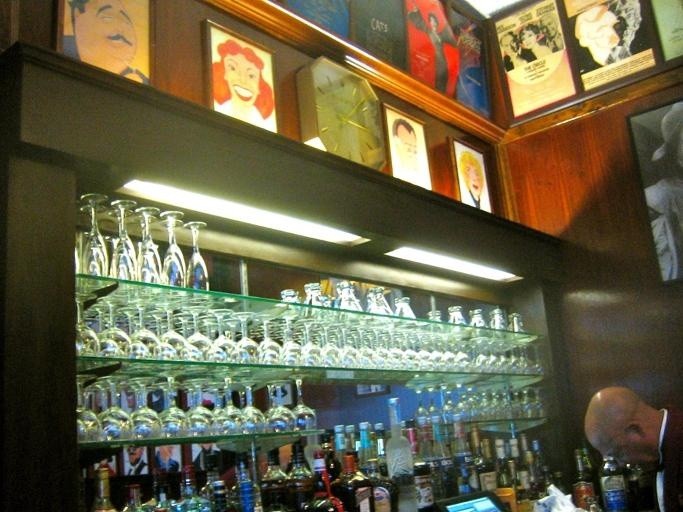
left=624, top=93, right=682, bottom=285
left=442, top=0, right=682, bottom=128
left=199, top=18, right=281, bottom=137
left=51, top=0, right=157, bottom=86
left=381, top=102, right=436, bottom=192
left=448, top=135, right=495, bottom=215
left=352, top=383, right=392, bottom=399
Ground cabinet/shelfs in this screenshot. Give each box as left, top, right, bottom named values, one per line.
left=75, top=272, right=547, bottom=451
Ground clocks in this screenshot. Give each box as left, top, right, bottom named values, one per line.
left=296, top=56, right=387, bottom=172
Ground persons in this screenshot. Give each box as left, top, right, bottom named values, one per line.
left=58, top=0, right=151, bottom=82
left=461, top=149, right=491, bottom=212
left=516, top=25, right=553, bottom=63
left=154, top=445, right=181, bottom=473
left=394, top=118, right=432, bottom=191
left=500, top=31, right=536, bottom=71
left=123, top=447, right=149, bottom=475
left=210, top=40, right=275, bottom=132
left=585, top=386, right=683, bottom=512
left=645, top=98, right=682, bottom=282
left=536, top=13, right=563, bottom=52
left=411, top=4, right=456, bottom=94
left=192, top=443, right=220, bottom=472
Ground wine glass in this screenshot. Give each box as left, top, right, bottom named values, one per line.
left=80, top=192, right=209, bottom=291
left=74, top=293, right=545, bottom=374
left=77, top=377, right=546, bottom=441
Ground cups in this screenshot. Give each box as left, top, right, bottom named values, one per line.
left=281, top=280, right=531, bottom=333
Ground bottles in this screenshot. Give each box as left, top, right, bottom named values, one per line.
left=91, top=399, right=639, bottom=512
